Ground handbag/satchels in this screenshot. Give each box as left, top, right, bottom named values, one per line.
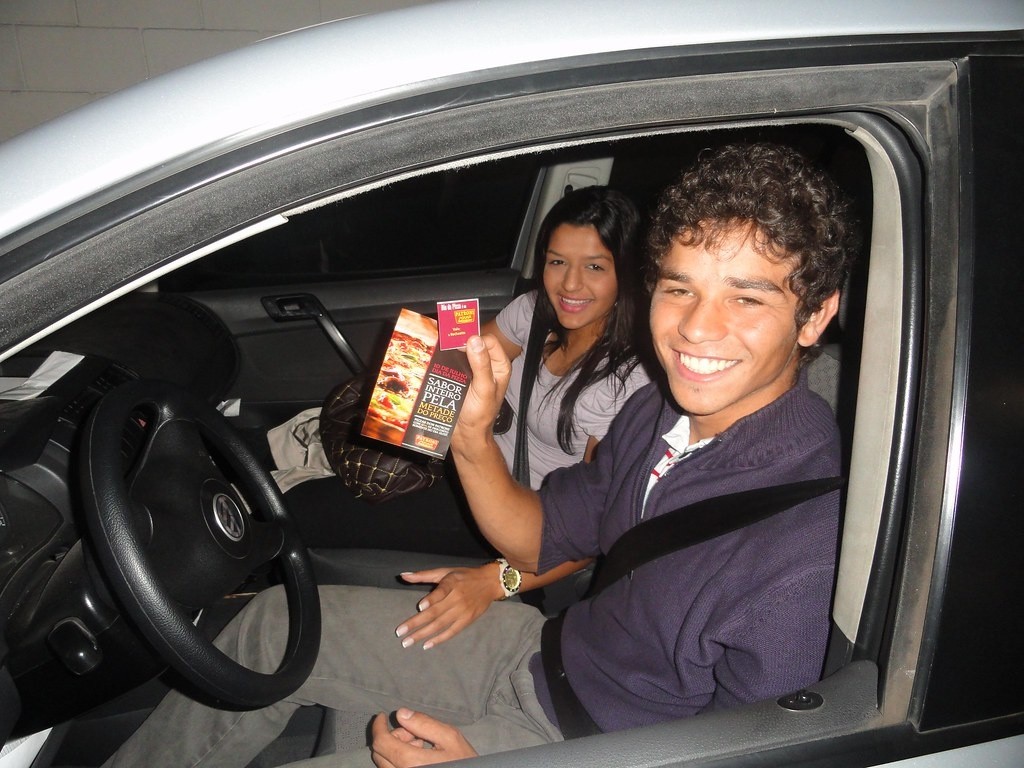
left=320, top=374, right=435, bottom=505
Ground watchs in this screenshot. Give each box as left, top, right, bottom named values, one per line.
left=496, top=558, right=522, bottom=601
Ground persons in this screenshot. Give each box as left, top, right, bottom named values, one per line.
left=254, top=183, right=655, bottom=650
left=98, top=140, right=851, bottom=768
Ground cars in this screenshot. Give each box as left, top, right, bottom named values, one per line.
left=2, top=4, right=1024, bottom=768
